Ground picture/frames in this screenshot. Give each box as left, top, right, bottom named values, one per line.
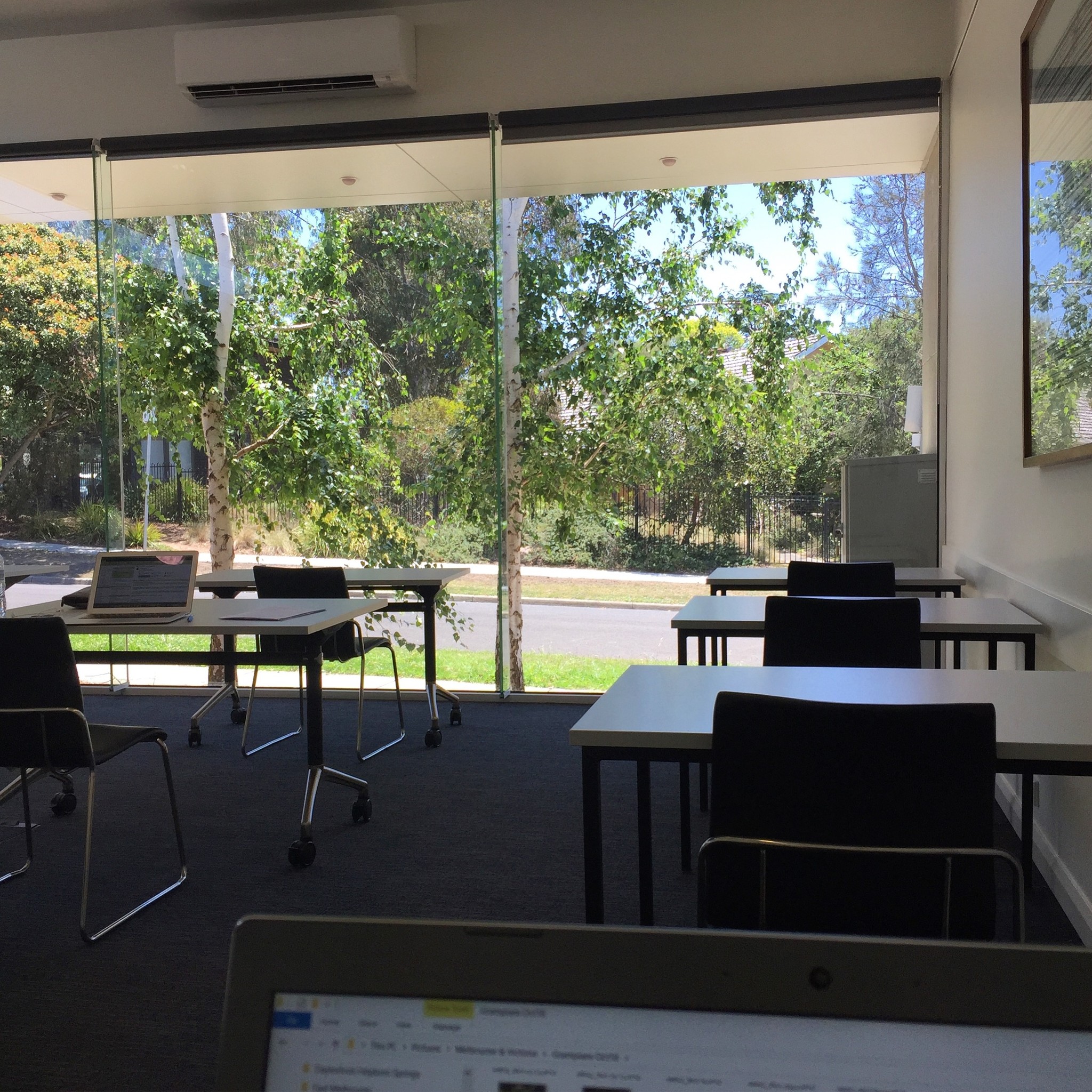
left=1019, top=0, right=1092, bottom=471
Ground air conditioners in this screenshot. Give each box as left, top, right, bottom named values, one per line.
left=174, top=15, right=418, bottom=109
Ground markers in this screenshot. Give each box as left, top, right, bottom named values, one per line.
left=187, top=614, right=194, bottom=622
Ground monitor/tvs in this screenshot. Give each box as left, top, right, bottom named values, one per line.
left=213, top=916, right=1092, bottom=1092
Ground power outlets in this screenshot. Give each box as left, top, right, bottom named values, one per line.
left=1032, top=780, right=1039, bottom=809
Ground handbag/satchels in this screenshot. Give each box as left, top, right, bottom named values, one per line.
left=61, top=587, right=92, bottom=609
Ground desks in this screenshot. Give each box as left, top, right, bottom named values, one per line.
left=705, top=567, right=966, bottom=669
left=4, top=565, right=69, bottom=591
left=188, top=567, right=471, bottom=749
left=671, top=594, right=1046, bottom=670
left=566, top=664, right=1092, bottom=927
left=0, top=599, right=389, bottom=869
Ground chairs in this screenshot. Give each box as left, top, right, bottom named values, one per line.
left=0, top=616, right=189, bottom=946
left=786, top=561, right=896, bottom=597
left=761, top=595, right=923, bottom=670
left=694, top=691, right=1030, bottom=944
left=240, top=566, right=407, bottom=763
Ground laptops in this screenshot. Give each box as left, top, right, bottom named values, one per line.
left=64, top=550, right=199, bottom=625
